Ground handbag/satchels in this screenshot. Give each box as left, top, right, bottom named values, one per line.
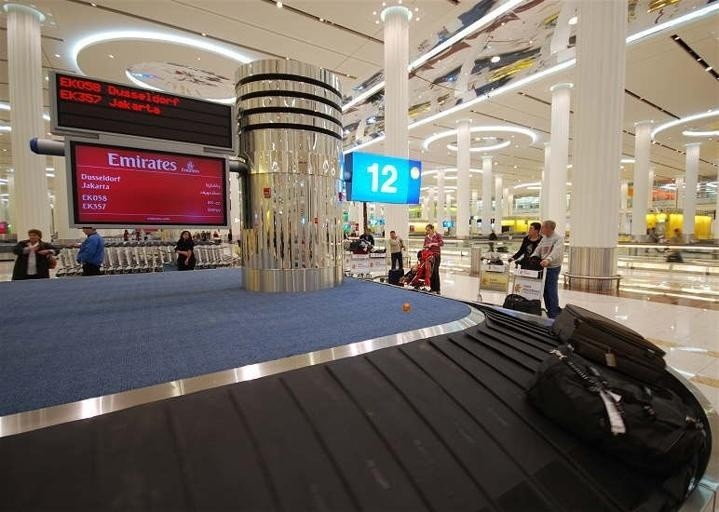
left=525, top=346, right=708, bottom=475
left=503, top=294, right=541, bottom=316
left=46, top=256, right=56, bottom=269
left=522, top=256, right=543, bottom=278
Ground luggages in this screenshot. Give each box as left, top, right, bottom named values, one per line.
left=553, top=304, right=666, bottom=383
left=389, top=268, right=403, bottom=287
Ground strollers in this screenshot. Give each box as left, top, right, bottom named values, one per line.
left=399, top=245, right=437, bottom=292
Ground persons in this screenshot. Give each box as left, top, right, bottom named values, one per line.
left=76, top=228, right=106, bottom=275
left=11, top=229, right=60, bottom=280
left=488, top=230, right=498, bottom=252
left=668, top=227, right=686, bottom=263
left=360, top=230, right=375, bottom=245
left=530, top=221, right=566, bottom=317
left=192, top=228, right=231, bottom=242
left=175, top=231, right=196, bottom=270
left=123, top=229, right=152, bottom=242
left=508, top=222, right=543, bottom=279
left=388, top=231, right=407, bottom=272
left=399, top=250, right=422, bottom=285
left=644, top=227, right=664, bottom=253
left=423, top=224, right=444, bottom=294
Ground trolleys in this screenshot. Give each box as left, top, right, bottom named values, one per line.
left=343, top=244, right=386, bottom=284
left=55, top=242, right=240, bottom=277
left=476, top=255, right=548, bottom=308
